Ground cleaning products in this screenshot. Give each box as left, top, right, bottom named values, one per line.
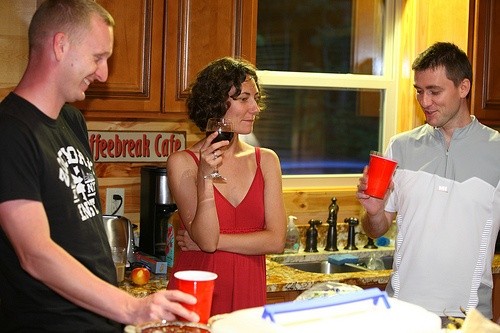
left=283, top=215, right=300, bottom=254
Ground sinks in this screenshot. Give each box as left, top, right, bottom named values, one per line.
left=280, top=259, right=367, bottom=274
left=356, top=256, right=394, bottom=271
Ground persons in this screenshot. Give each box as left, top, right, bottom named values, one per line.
left=166, top=57, right=288, bottom=317
left=0, top=0, right=200, bottom=333
left=357, top=42, right=500, bottom=329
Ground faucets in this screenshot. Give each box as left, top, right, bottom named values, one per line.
left=324, top=196, right=339, bottom=251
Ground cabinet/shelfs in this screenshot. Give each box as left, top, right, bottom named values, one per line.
left=416, top=0, right=500, bottom=128
left=37, top=0, right=258, bottom=122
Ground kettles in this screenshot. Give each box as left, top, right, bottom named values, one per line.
left=103, top=215, right=136, bottom=265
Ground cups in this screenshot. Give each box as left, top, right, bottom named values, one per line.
left=111, top=247, right=128, bottom=286
left=363, top=153, right=399, bottom=200
left=173, top=270, right=218, bottom=325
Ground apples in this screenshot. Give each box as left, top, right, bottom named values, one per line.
left=131, top=267, right=150, bottom=284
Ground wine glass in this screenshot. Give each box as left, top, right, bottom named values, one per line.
left=202, top=117, right=234, bottom=184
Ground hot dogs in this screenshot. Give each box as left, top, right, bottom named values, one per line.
left=135, top=319, right=212, bottom=333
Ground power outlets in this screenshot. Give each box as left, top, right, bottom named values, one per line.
left=105, top=188, right=125, bottom=216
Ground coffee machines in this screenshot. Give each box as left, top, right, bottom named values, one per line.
left=140, top=165, right=177, bottom=261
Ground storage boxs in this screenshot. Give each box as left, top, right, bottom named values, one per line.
left=135, top=251, right=167, bottom=274
